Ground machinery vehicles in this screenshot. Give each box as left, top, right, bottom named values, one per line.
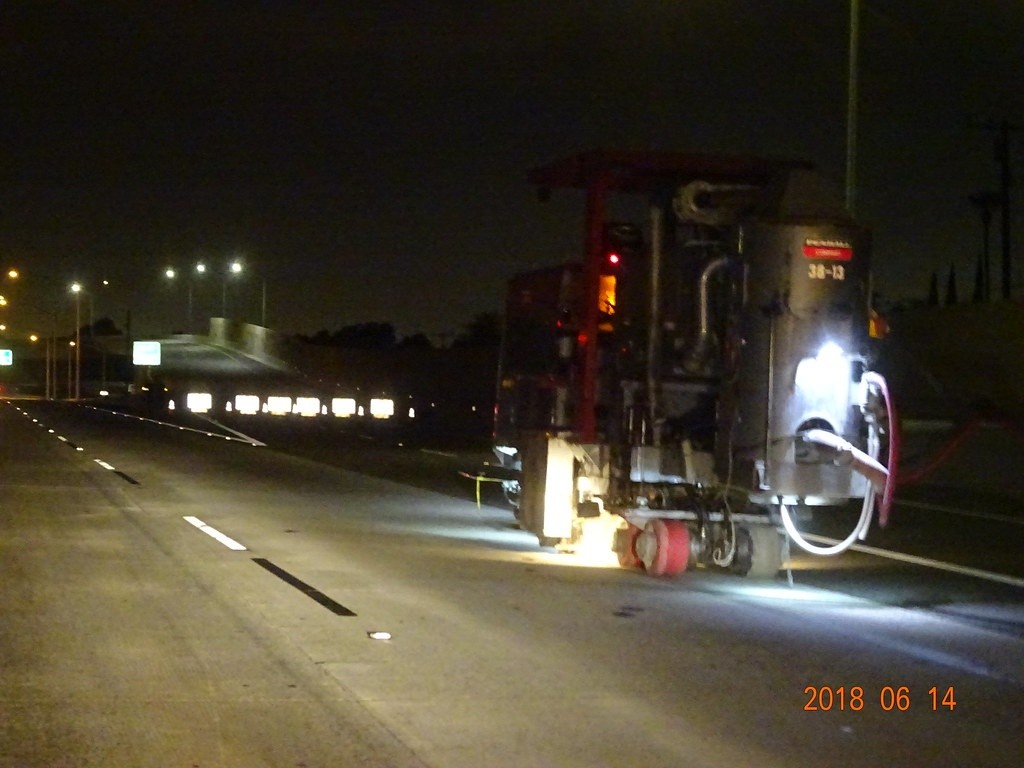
left=455, top=152, right=899, bottom=576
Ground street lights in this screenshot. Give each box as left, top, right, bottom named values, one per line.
left=198, top=264, right=226, bottom=319
left=232, top=263, right=267, bottom=328
left=71, top=284, right=132, bottom=388
left=0, top=321, right=50, bottom=399
left=0, top=299, right=57, bottom=398
left=166, top=270, right=193, bottom=315
left=9, top=268, right=80, bottom=398
